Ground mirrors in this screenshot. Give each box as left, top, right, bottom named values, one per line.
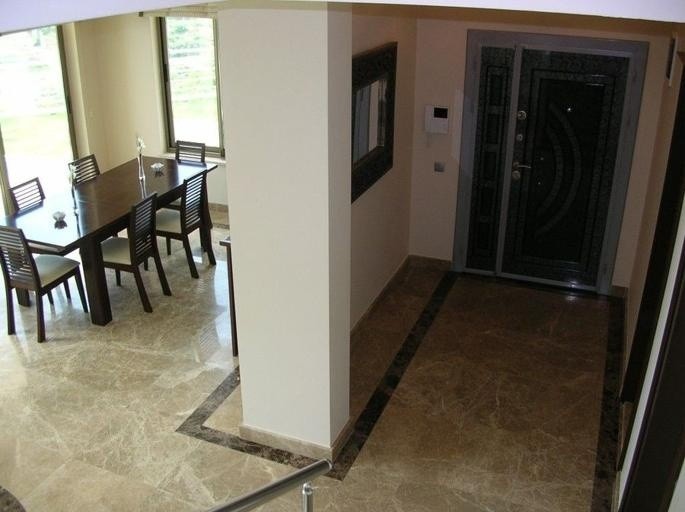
left=351, top=41, right=398, bottom=206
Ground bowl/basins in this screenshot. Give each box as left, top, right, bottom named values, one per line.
left=150, top=162, right=164, bottom=172
left=53, top=211, right=66, bottom=222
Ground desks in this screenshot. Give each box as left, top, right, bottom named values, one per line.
left=219, top=234, right=238, bottom=357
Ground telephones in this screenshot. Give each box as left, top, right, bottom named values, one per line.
left=424, top=103, right=449, bottom=134
